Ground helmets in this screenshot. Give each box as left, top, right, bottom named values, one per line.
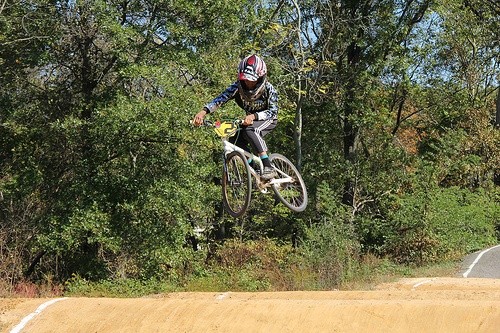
left=237, top=54, right=268, bottom=102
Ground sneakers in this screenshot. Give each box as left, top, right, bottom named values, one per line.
left=253, top=167, right=261, bottom=189
left=263, top=166, right=278, bottom=179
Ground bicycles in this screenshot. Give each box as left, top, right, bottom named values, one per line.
left=189, top=116, right=308, bottom=218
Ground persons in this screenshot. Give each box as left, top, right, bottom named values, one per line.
left=192, top=53, right=280, bottom=182
